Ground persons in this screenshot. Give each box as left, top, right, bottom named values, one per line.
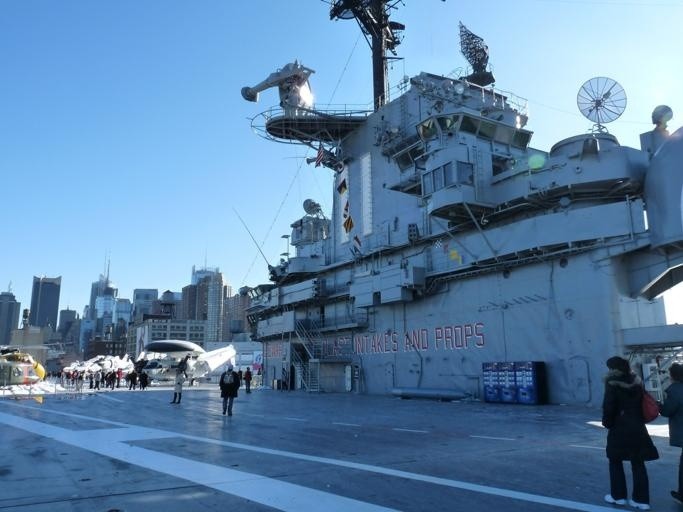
left=242, top=366, right=252, bottom=393
left=602, top=356, right=660, bottom=510
left=42, top=365, right=151, bottom=392
left=218, top=364, right=240, bottom=416
left=170, top=363, right=184, bottom=406
left=656, top=363, right=682, bottom=505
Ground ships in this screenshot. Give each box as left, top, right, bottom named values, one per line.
left=240, top=1, right=683, bottom=414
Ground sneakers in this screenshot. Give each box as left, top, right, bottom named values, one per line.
left=670, top=490, right=683, bottom=505
left=629, top=499, right=651, bottom=510
left=605, top=494, right=626, bottom=505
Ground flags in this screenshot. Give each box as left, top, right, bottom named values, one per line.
left=336, top=178, right=347, bottom=195
left=351, top=235, right=361, bottom=254
left=454, top=255, right=464, bottom=264
left=434, top=238, right=443, bottom=250
left=343, top=201, right=352, bottom=217
left=341, top=217, right=353, bottom=234
left=314, top=144, right=326, bottom=167
left=449, top=250, right=457, bottom=260
left=441, top=244, right=449, bottom=251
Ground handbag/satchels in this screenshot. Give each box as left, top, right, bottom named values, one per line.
left=643, top=393, right=660, bottom=424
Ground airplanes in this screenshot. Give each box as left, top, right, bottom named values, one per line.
left=138, top=340, right=237, bottom=384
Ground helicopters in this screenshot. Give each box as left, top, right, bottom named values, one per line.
left=0, top=343, right=57, bottom=401
